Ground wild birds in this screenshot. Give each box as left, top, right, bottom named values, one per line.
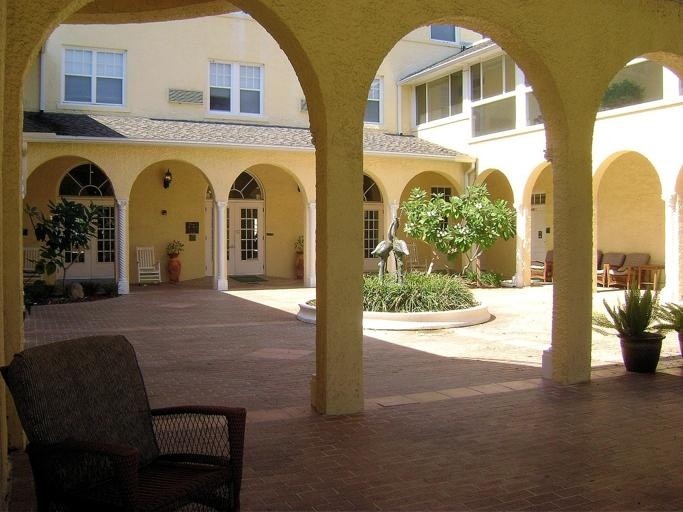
left=369, top=215, right=410, bottom=284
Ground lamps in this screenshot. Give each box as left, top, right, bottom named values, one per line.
left=163, top=168, right=172, bottom=190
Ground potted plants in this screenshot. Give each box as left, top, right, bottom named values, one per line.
left=166, top=239, right=186, bottom=283
left=593, top=273, right=681, bottom=376
left=293, top=231, right=305, bottom=280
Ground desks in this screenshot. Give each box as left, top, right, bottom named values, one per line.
left=637, top=262, right=665, bottom=291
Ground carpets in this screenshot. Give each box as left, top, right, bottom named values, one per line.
left=230, top=273, right=267, bottom=285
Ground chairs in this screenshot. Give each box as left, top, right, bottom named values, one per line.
left=530, top=248, right=651, bottom=291
left=1, top=333, right=247, bottom=512
left=135, top=245, right=162, bottom=289
